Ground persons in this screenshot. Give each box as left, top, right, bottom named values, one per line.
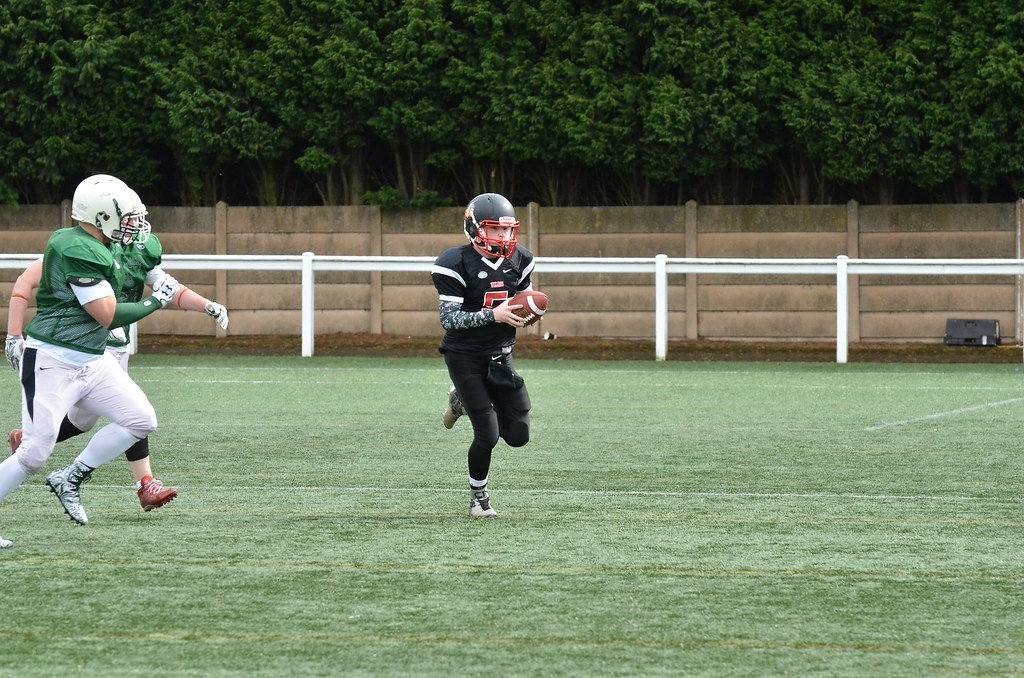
left=431, top=192, right=543, bottom=517
left=0, top=174, right=179, bottom=549
left=5, top=188, right=228, bottom=513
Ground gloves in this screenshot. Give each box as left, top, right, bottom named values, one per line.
left=5, top=335, right=25, bottom=371
left=205, top=301, right=229, bottom=330
left=152, top=274, right=180, bottom=308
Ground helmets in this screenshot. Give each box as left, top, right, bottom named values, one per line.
left=129, top=187, right=147, bottom=215
left=71, top=174, right=138, bottom=241
left=464, top=193, right=516, bottom=255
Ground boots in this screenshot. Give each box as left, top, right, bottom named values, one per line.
left=45, top=461, right=97, bottom=526
left=0, top=537, right=12, bottom=549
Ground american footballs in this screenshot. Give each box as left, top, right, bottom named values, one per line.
left=508, top=290, right=548, bottom=328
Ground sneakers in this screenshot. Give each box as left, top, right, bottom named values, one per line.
left=442, top=385, right=468, bottom=429
left=469, top=491, right=497, bottom=518
left=138, top=475, right=178, bottom=511
left=7, top=429, right=22, bottom=455
